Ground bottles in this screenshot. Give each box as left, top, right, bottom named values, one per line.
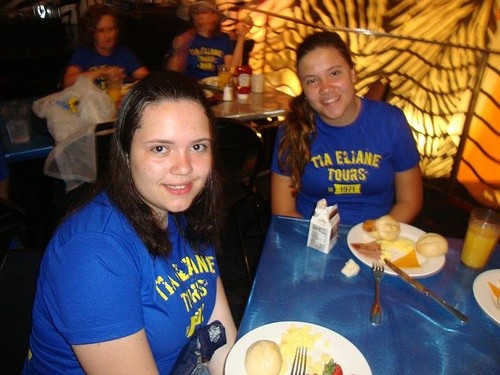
left=235, top=66, right=252, bottom=101
left=222, top=83, right=234, bottom=101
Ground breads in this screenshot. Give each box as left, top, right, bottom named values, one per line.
left=375, top=215, right=401, bottom=240
left=244, top=339, right=282, bottom=375
left=415, top=232, right=449, bottom=256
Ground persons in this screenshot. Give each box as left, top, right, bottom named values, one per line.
left=25, top=69, right=237, bottom=375
left=271, top=29, right=424, bottom=225
left=168, top=2, right=262, bottom=191
left=62, top=3, right=149, bottom=86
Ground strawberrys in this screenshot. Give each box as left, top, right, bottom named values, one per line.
left=322, top=359, right=343, bottom=375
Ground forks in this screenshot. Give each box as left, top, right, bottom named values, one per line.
left=290, top=346, right=307, bottom=375
left=370, top=261, right=384, bottom=326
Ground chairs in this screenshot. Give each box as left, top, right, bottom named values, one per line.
left=212, top=116, right=280, bottom=286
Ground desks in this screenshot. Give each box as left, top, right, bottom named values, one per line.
left=198, top=76, right=294, bottom=121
left=0, top=100, right=54, bottom=161
left=236, top=214, right=500, bottom=375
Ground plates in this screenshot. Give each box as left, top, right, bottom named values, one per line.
left=346, top=221, right=446, bottom=278
left=472, top=269, right=500, bottom=323
left=201, top=77, right=217, bottom=85
left=224, top=321, right=372, bottom=375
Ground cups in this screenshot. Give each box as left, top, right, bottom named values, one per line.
left=217, top=65, right=231, bottom=86
left=459, top=209, right=500, bottom=271
left=3, top=108, right=29, bottom=143
left=250, top=75, right=264, bottom=94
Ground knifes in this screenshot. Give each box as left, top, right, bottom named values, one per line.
left=384, top=257, right=468, bottom=322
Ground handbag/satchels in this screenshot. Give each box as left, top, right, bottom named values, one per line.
left=170, top=320, right=227, bottom=375
